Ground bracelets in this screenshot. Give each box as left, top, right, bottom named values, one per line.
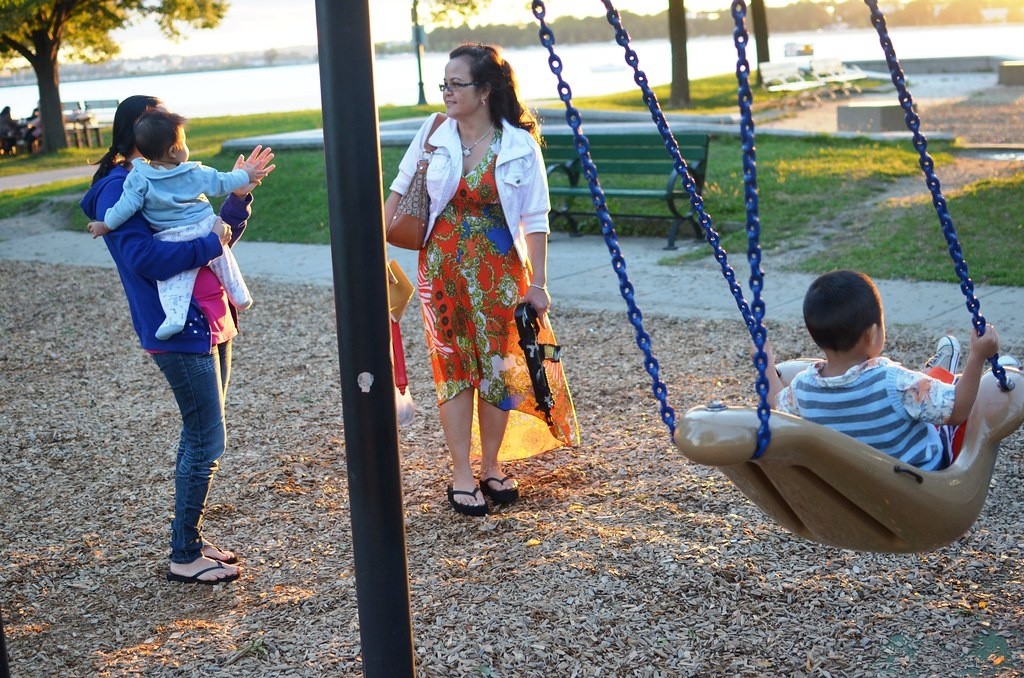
left=530, top=283, right=546, bottom=291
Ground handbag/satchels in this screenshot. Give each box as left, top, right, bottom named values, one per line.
left=385, top=112, right=447, bottom=250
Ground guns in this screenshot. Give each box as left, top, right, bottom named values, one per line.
left=514, top=302, right=564, bottom=428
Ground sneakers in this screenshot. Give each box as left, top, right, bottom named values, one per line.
left=997, top=354, right=1022, bottom=368
left=924, top=335, right=961, bottom=375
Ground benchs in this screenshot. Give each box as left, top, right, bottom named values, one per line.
left=84, top=100, right=119, bottom=121
left=538, top=134, right=711, bottom=251
left=757, top=58, right=825, bottom=120
left=60, top=102, right=81, bottom=123
left=809, top=57, right=869, bottom=100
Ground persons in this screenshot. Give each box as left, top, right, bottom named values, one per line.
left=0, top=106, right=26, bottom=156
left=24, top=107, right=43, bottom=154
left=87, top=108, right=266, bottom=341
left=80, top=95, right=278, bottom=584
left=752, top=271, right=1021, bottom=471
left=383, top=45, right=581, bottom=517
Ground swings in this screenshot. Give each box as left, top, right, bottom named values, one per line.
left=528, top=0, right=1023, bottom=554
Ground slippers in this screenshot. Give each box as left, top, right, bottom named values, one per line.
left=480, top=476, right=521, bottom=501
left=447, top=488, right=488, bottom=517
left=200, top=543, right=239, bottom=564
left=166, top=558, right=241, bottom=584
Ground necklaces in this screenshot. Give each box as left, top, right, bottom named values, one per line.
left=460, top=121, right=494, bottom=157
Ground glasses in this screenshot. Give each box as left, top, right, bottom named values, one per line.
left=439, top=81, right=481, bottom=92
